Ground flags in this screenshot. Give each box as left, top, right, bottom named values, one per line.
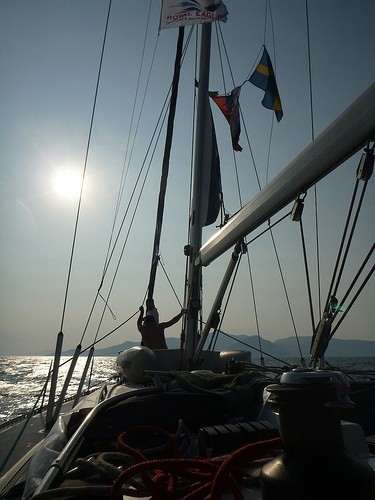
left=249, top=47, right=285, bottom=122
left=160, top=0, right=227, bottom=33
left=211, top=79, right=247, bottom=152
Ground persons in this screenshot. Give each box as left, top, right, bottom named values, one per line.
left=145, top=300, right=160, bottom=322
left=138, top=305, right=185, bottom=349
